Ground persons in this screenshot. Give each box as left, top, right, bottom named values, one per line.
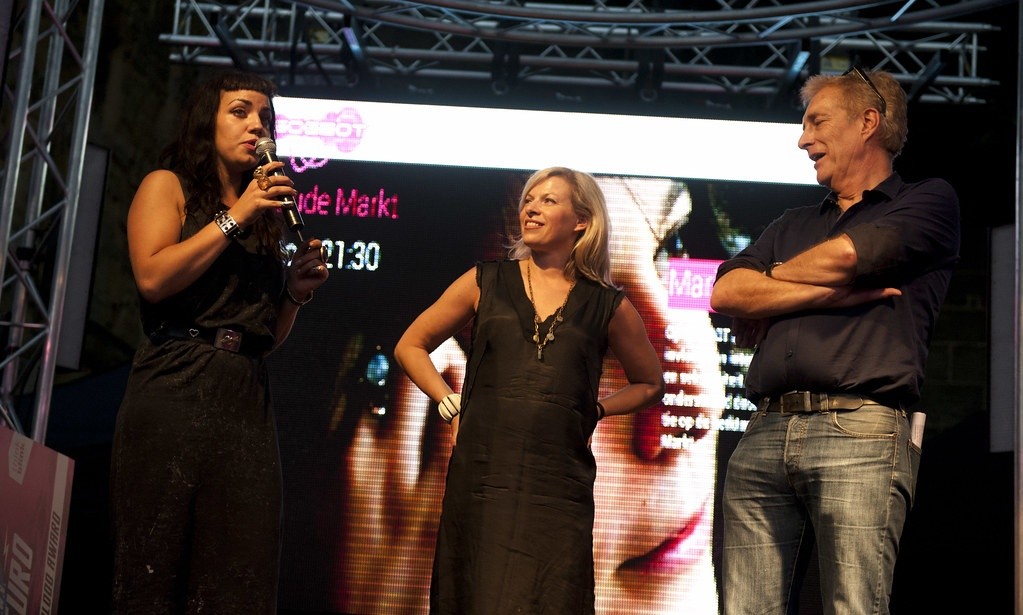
left=392, top=165, right=666, bottom=615
left=709, top=71, right=961, bottom=615
left=111, top=68, right=329, bottom=615
left=276, top=156, right=725, bottom=615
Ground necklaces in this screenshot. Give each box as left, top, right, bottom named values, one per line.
left=527, top=259, right=574, bottom=360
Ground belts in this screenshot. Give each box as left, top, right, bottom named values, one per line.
left=753, top=390, right=879, bottom=412
left=149, top=320, right=267, bottom=361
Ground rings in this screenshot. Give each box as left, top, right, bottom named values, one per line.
left=258, top=175, right=272, bottom=190
left=253, top=165, right=263, bottom=179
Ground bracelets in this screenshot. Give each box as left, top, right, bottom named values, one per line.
left=437, top=393, right=461, bottom=423
left=286, top=288, right=313, bottom=306
left=597, top=401, right=604, bottom=421
left=214, top=210, right=244, bottom=241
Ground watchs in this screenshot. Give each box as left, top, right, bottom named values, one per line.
left=766, top=262, right=783, bottom=277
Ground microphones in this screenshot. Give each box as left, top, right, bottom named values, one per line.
left=254, top=137, right=305, bottom=244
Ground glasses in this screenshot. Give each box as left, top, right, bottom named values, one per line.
left=841, top=63, right=888, bottom=116
